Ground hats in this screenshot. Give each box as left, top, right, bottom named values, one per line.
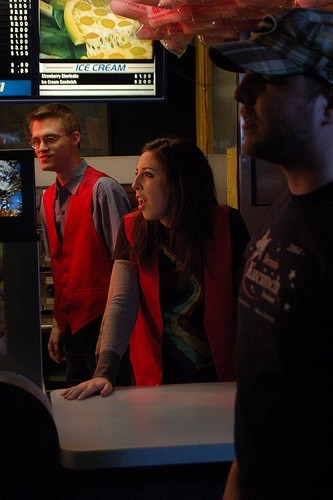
left=208, top=8, right=333, bottom=83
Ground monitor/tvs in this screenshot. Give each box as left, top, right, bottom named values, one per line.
left=0, top=0, right=167, bottom=101
left=0, top=148, right=37, bottom=242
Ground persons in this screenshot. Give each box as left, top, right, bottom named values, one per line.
left=25, top=103, right=131, bottom=388
left=61, top=137, right=252, bottom=400
left=207, top=9, right=332, bottom=500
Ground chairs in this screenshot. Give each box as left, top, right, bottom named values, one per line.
left=0, top=372, right=61, bottom=500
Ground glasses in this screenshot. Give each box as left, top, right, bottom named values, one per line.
left=28, top=134, right=67, bottom=147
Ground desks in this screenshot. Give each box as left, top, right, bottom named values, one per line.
left=50, top=380, right=237, bottom=500
